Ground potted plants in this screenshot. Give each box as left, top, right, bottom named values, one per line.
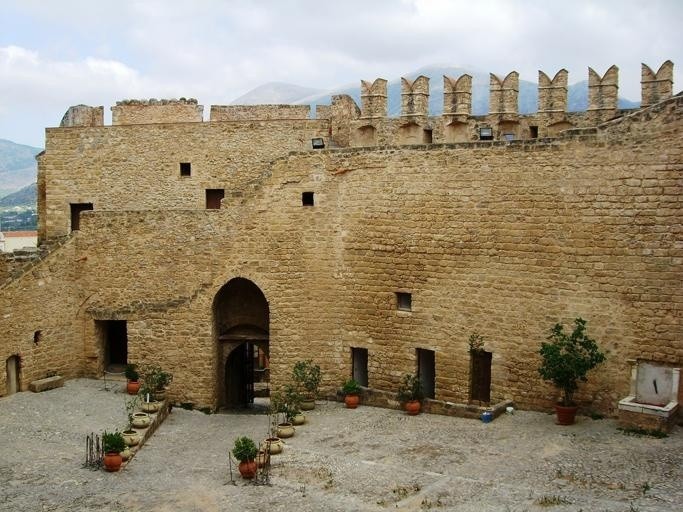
left=341, top=379, right=363, bottom=409
left=395, top=374, right=425, bottom=415
left=101, top=364, right=174, bottom=472
left=231, top=358, right=324, bottom=480
left=536, top=316, right=607, bottom=425
